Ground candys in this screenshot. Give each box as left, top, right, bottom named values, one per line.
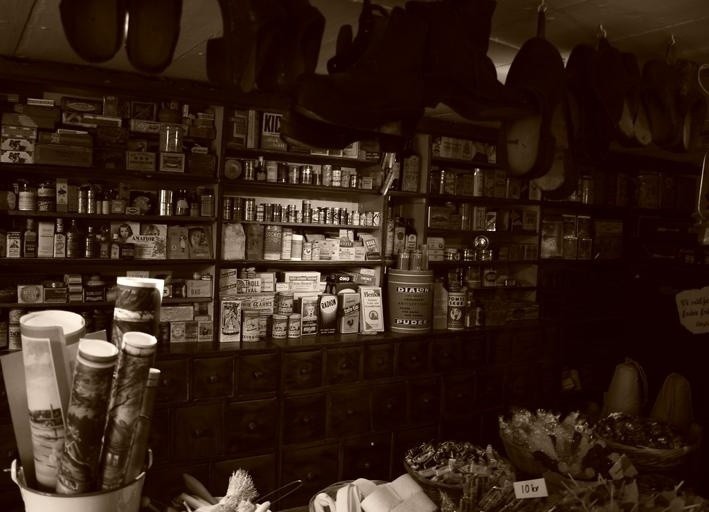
left=404, top=409, right=709, bottom=512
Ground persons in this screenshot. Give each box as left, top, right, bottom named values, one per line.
left=189, top=228, right=207, bottom=248
left=141, top=224, right=161, bottom=236
left=113, top=223, right=134, bottom=242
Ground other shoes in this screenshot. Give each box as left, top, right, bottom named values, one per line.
left=127, top=0, right=182, bottom=67
left=335, top=1, right=702, bottom=200
left=208, top=2, right=324, bottom=109
left=61, top=1, right=125, bottom=63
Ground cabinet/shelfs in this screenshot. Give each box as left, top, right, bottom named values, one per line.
left=217, top=97, right=390, bottom=492
left=2, top=68, right=226, bottom=512
left=387, top=114, right=543, bottom=493
left=545, top=135, right=703, bottom=483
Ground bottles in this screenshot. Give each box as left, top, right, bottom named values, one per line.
left=473, top=167, right=483, bottom=196
left=5, top=180, right=214, bottom=261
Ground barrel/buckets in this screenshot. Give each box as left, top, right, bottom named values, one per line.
left=387, top=268, right=433, bottom=334
left=11, top=448, right=153, bottom=512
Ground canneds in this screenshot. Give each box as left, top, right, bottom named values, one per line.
left=241, top=156, right=372, bottom=190
left=18, top=184, right=56, bottom=212
left=200, top=195, right=215, bottom=217
left=395, top=249, right=422, bottom=271
left=242, top=310, right=261, bottom=343
left=159, top=189, right=173, bottom=216
left=222, top=195, right=380, bottom=227
left=161, top=125, right=183, bottom=153
left=445, top=292, right=467, bottom=330
